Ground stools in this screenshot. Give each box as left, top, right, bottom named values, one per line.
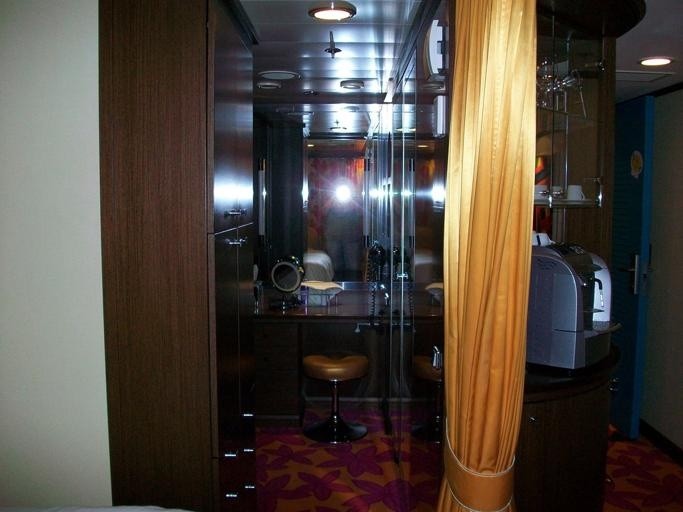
left=300, top=350, right=370, bottom=442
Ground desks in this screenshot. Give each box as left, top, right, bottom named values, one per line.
left=257, top=291, right=442, bottom=429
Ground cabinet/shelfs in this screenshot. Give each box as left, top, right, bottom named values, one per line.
left=101, top=0, right=260, bottom=512
left=409, top=1, right=648, bottom=511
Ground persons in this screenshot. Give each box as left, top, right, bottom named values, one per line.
left=326, top=208, right=360, bottom=271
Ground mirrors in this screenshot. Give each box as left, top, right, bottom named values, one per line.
left=254, top=100, right=452, bottom=295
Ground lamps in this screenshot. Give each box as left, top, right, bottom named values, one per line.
left=306, top=2, right=358, bottom=26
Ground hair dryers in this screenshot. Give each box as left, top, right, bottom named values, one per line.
left=371, top=246, right=386, bottom=281
left=394, top=247, right=411, bottom=281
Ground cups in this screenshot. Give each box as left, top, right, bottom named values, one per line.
left=534, top=184, right=586, bottom=200
